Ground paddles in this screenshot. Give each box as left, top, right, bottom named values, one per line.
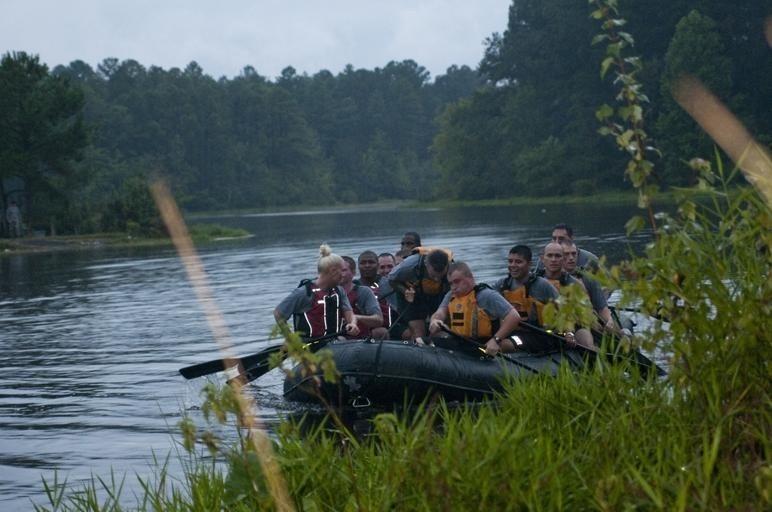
left=608, top=304, right=712, bottom=324
left=573, top=323, right=668, bottom=375
left=179, top=329, right=355, bottom=387
left=519, top=320, right=658, bottom=383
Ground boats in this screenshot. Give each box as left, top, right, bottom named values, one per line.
left=277, top=323, right=666, bottom=424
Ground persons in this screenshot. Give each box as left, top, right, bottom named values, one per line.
left=387, top=245, right=454, bottom=345
left=275, top=232, right=422, bottom=351
left=492, top=226, right=632, bottom=348
left=429, top=262, right=519, bottom=357
